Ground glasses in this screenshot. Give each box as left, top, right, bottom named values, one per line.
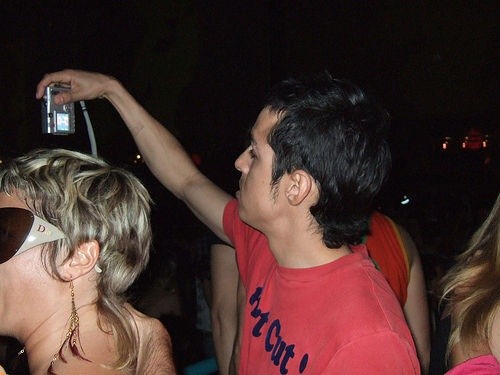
left=0, top=207, right=104, bottom=276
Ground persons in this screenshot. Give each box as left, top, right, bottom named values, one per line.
left=431, top=193, right=500, bottom=375
left=210, top=210, right=431, bottom=373
left=0, top=147, right=154, bottom=375
left=35, top=69, right=421, bottom=374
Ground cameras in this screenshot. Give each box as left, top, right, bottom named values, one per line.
left=41, top=86, right=75, bottom=135
